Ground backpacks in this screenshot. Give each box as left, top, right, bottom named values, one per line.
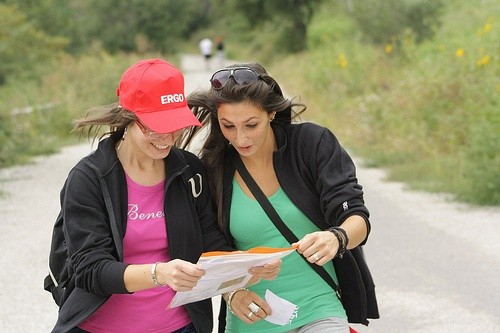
left=41, top=145, right=197, bottom=308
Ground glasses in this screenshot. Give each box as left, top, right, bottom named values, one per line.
left=209, top=67, right=270, bottom=90
left=133, top=118, right=157, bottom=137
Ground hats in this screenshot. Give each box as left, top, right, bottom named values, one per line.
left=117, top=59, right=202, bottom=133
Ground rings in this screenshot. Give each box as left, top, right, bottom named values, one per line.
left=247, top=312, right=253, bottom=318
left=248, top=302, right=260, bottom=314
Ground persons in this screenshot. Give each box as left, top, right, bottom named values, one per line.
left=214, top=36, right=226, bottom=71
left=198, top=35, right=213, bottom=72
left=186, top=62, right=382, bottom=333
left=42, top=56, right=283, bottom=333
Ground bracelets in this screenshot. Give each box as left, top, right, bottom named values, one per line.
left=227, top=287, right=250, bottom=316
left=151, top=261, right=163, bottom=286
left=327, top=227, right=351, bottom=259
